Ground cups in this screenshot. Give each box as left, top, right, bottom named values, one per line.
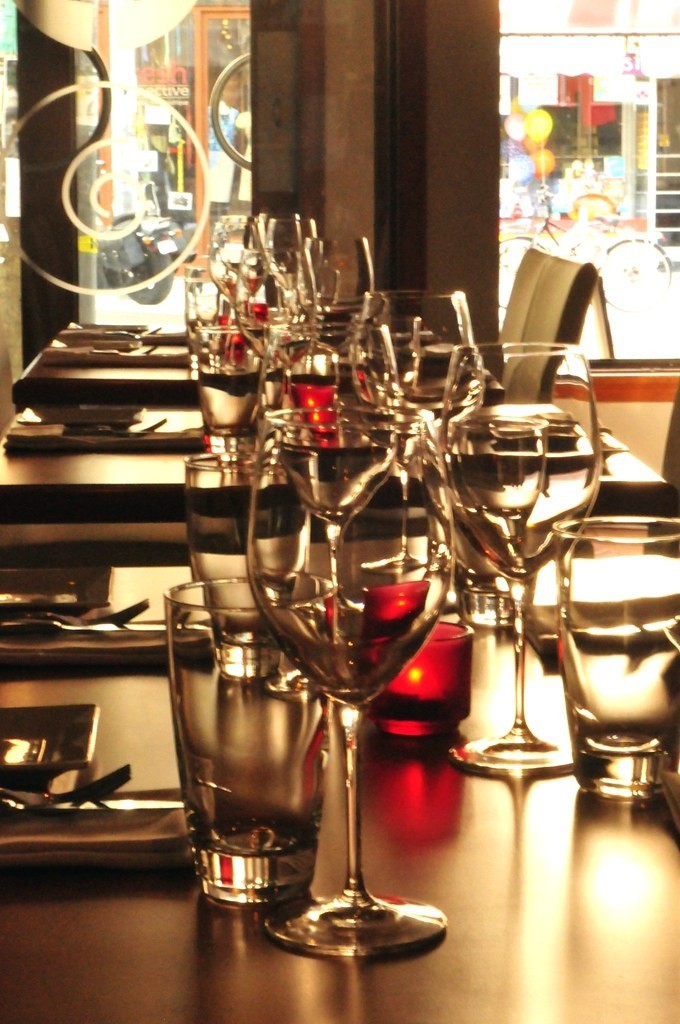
left=163, top=574, right=342, bottom=909
left=183, top=452, right=309, bottom=685
left=552, top=514, right=680, bottom=803
left=453, top=556, right=536, bottom=629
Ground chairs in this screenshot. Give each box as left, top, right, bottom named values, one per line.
left=491, top=248, right=603, bottom=400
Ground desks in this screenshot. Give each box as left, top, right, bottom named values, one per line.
left=0, top=320, right=680, bottom=1022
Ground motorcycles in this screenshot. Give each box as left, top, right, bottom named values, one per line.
left=99, top=208, right=182, bottom=305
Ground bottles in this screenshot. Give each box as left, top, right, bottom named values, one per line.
left=353, top=583, right=475, bottom=738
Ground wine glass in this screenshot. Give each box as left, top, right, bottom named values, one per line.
left=183, top=208, right=484, bottom=614
left=247, top=406, right=455, bottom=961
left=439, top=341, right=604, bottom=781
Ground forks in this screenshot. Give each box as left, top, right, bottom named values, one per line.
left=0, top=596, right=152, bottom=633
left=0, top=763, right=131, bottom=816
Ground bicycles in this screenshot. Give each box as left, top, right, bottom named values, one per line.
left=497, top=184, right=671, bottom=314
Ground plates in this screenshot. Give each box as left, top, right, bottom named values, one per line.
left=0, top=410, right=207, bottom=453
left=0, top=789, right=192, bottom=869
left=3, top=618, right=219, bottom=668
left=0, top=703, right=102, bottom=772
left=41, top=328, right=192, bottom=368
